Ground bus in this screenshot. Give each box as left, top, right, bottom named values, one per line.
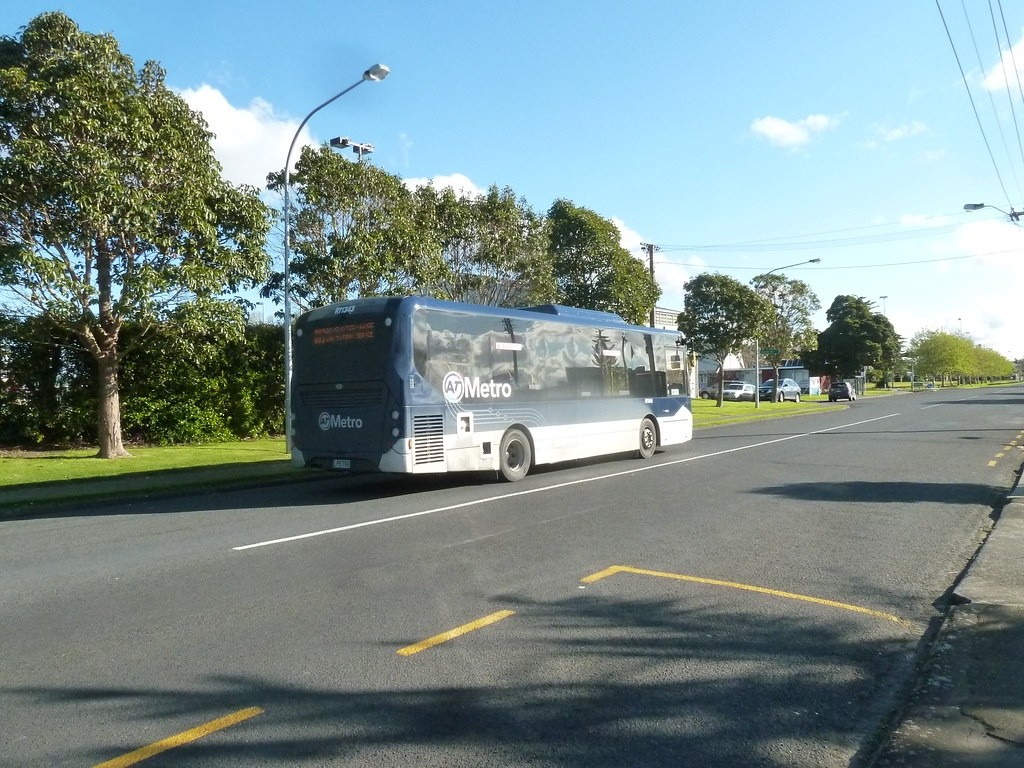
left=287, top=293, right=695, bottom=484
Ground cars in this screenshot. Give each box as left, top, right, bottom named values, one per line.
left=828, top=382, right=857, bottom=403
left=714, top=383, right=757, bottom=402
left=699, top=380, right=746, bottom=400
left=759, top=378, right=801, bottom=404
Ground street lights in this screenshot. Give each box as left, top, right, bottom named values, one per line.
left=283, top=61, right=392, bottom=464
left=754, top=257, right=821, bottom=410
left=880, top=296, right=888, bottom=318
